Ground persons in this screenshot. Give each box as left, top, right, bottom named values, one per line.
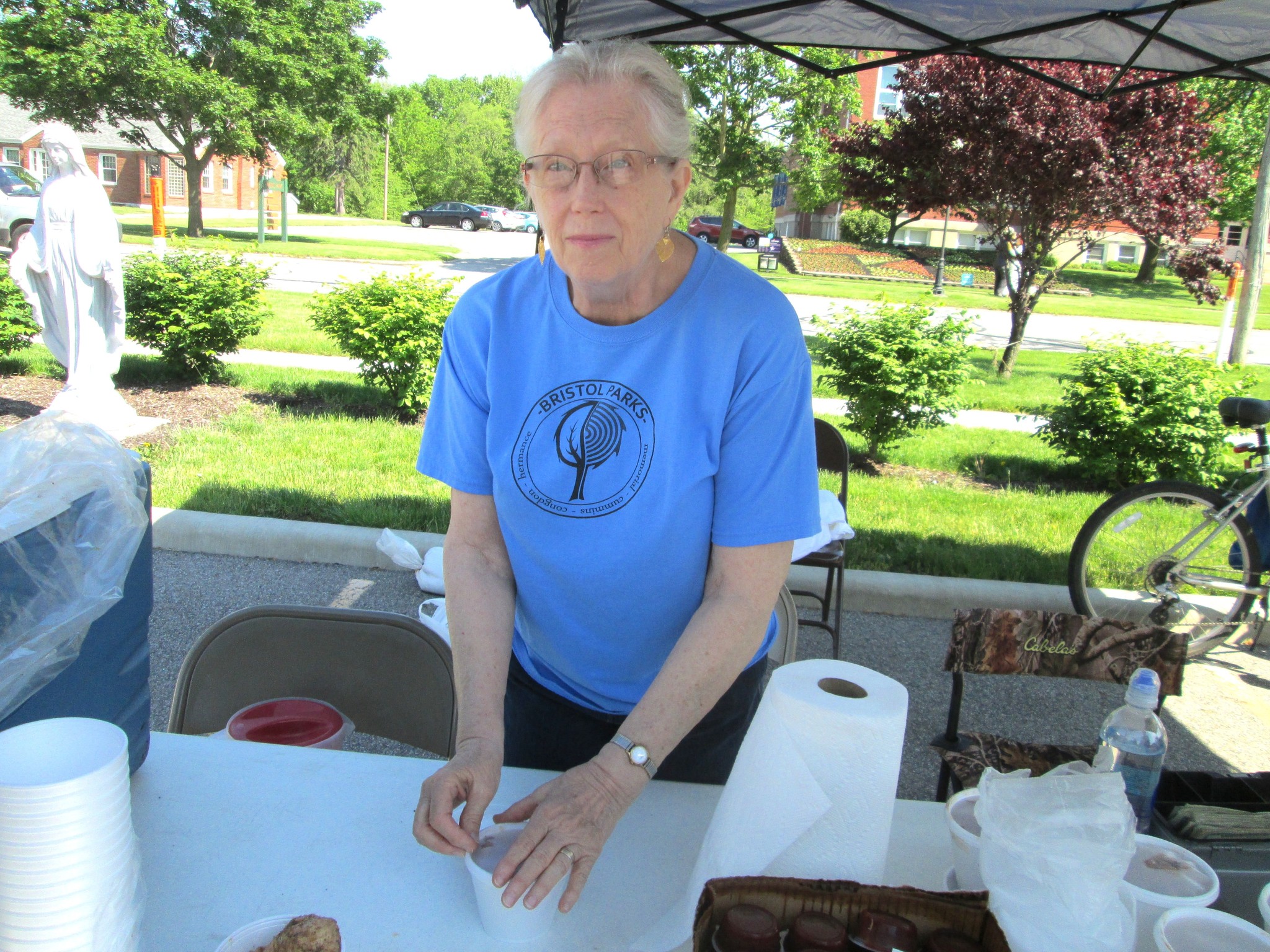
left=10, top=121, right=165, bottom=438
left=993, top=228, right=1025, bottom=298
left=416, top=40, right=824, bottom=915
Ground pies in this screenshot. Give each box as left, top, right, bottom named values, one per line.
left=249, top=914, right=342, bottom=952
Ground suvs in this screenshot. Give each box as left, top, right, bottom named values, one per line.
left=688, top=215, right=762, bottom=249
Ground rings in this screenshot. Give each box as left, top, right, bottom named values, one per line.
left=560, top=847, right=575, bottom=866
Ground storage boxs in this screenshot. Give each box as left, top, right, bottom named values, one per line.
left=1150, top=815, right=1270, bottom=931
left=0, top=433, right=153, bottom=776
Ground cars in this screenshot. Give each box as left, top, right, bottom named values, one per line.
left=400, top=201, right=494, bottom=232
left=511, top=210, right=539, bottom=233
left=0, top=161, right=44, bottom=254
left=473, top=205, right=525, bottom=232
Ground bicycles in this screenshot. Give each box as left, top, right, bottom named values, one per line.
left=1066, top=395, right=1270, bottom=661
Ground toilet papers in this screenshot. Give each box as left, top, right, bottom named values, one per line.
left=626, top=656, right=910, bottom=952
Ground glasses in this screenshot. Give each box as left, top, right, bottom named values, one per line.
left=520, top=150, right=678, bottom=189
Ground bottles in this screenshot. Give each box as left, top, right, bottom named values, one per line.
left=1092, top=668, right=1168, bottom=832
left=711, top=903, right=984, bottom=952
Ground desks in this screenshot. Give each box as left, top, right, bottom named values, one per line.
left=129, top=728, right=957, bottom=951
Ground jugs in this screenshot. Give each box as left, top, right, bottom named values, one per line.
left=209, top=697, right=356, bottom=751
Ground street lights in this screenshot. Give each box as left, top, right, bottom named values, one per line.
left=933, top=139, right=965, bottom=294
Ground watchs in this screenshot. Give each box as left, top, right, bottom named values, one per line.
left=610, top=734, right=658, bottom=780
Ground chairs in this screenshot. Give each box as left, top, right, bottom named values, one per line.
left=164, top=606, right=457, bottom=761
left=788, top=417, right=848, bottom=659
left=931, top=606, right=1191, bottom=804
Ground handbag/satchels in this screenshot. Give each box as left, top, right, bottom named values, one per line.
left=418, top=598, right=452, bottom=649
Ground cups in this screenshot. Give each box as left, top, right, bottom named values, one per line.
left=1151, top=885, right=1270, bottom=952
left=213, top=915, right=292, bottom=952
left=463, top=822, right=571, bottom=941
left=946, top=787, right=988, bottom=891
left=0, top=716, right=138, bottom=952
left=1119, top=832, right=1221, bottom=933
